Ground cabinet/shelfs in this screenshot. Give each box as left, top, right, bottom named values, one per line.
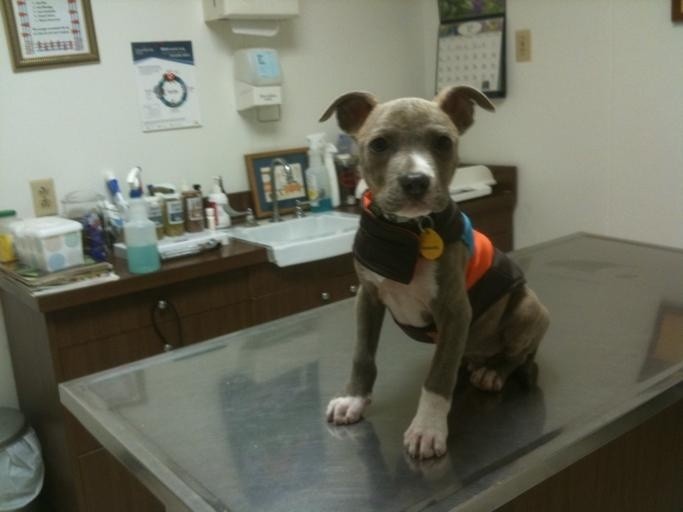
left=0, top=262, right=253, bottom=512
left=501, top=397, right=682, bottom=510
left=459, top=211, right=515, bottom=248
left=253, top=250, right=358, bottom=319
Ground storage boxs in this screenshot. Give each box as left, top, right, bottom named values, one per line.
left=9, top=215, right=83, bottom=271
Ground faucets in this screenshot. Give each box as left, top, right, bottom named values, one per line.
left=268, top=156, right=293, bottom=225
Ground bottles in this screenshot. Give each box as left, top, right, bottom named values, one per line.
left=152, top=183, right=205, bottom=235
left=335, top=152, right=357, bottom=205
left=205, top=207, right=218, bottom=229
left=0, top=209, right=24, bottom=263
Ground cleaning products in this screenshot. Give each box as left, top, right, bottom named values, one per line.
left=122, top=165, right=161, bottom=276
left=303, top=131, right=333, bottom=214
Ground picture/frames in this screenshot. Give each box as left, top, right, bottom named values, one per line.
left=245, top=146, right=314, bottom=219
left=0, top=0, right=99, bottom=72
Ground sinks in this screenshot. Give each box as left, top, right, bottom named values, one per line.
left=228, top=214, right=363, bottom=268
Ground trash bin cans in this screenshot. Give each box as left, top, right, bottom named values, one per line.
left=0, top=406, right=43, bottom=512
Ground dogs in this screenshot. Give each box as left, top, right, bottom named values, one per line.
left=317, top=84, right=550, bottom=463
